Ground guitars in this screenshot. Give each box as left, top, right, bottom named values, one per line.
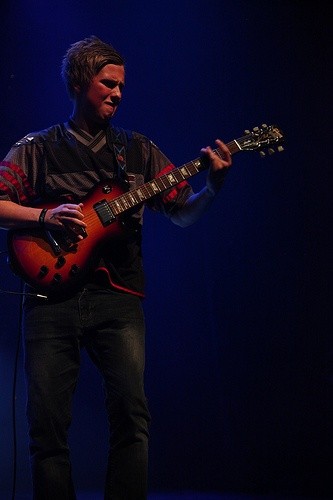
left=7, top=123, right=285, bottom=290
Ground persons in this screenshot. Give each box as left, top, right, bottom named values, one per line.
left=0, top=35, right=232, bottom=500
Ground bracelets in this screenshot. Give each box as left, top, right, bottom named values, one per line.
left=39, top=208, right=49, bottom=226
